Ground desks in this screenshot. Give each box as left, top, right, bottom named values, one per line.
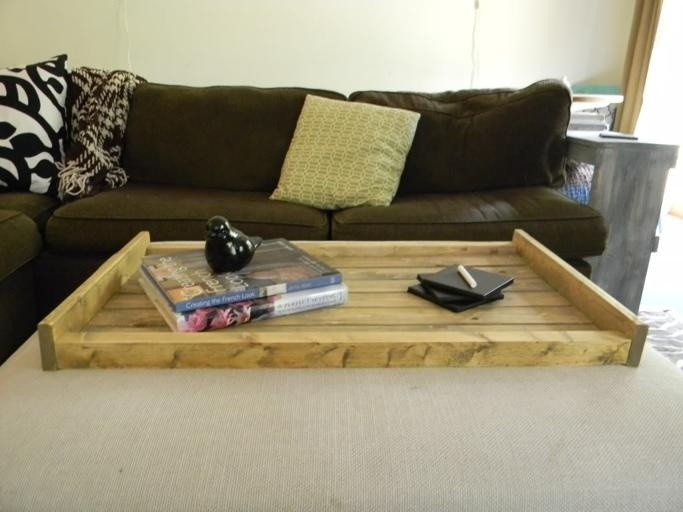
left=566, top=130, right=680, bottom=316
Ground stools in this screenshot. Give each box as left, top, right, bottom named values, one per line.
left=0, top=329, right=683, bottom=512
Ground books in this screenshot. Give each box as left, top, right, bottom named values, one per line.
left=138, top=267, right=347, bottom=332
left=138, top=237, right=342, bottom=313
left=569, top=85, right=624, bottom=132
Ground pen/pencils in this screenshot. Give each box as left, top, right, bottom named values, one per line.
left=458, top=265, right=477, bottom=288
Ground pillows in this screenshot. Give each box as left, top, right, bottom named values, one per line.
left=0, top=54, right=70, bottom=197
left=268, top=94, right=421, bottom=211
left=348, top=78, right=572, bottom=194
left=120, top=84, right=348, bottom=192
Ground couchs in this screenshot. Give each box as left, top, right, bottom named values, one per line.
left=0, top=89, right=606, bottom=367
